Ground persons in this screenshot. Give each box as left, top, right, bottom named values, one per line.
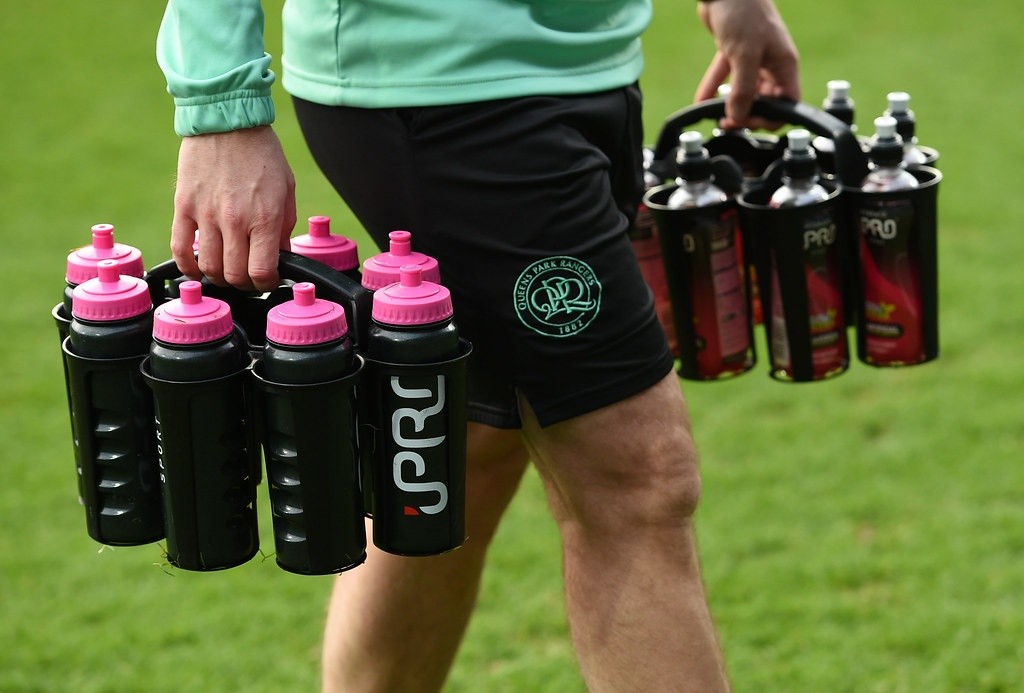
left=155, top=0, right=800, bottom=693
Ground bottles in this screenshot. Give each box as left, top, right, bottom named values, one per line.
left=62, top=217, right=459, bottom=387
left=641, top=78, right=928, bottom=210
